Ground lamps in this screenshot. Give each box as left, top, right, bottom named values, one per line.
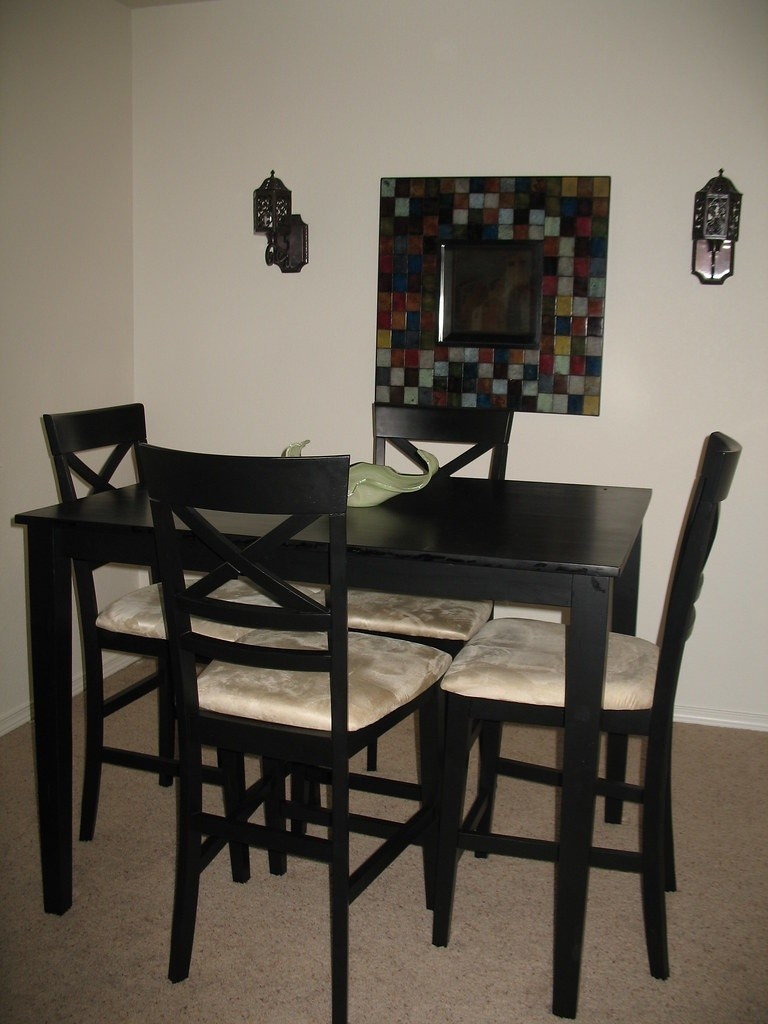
left=692, top=168, right=743, bottom=285
left=253, top=170, right=308, bottom=273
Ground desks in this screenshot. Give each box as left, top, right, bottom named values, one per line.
left=16, top=475, right=653, bottom=1019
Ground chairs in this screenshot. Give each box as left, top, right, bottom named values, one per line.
left=134, top=440, right=455, bottom=1024
left=307, top=403, right=515, bottom=772
left=431, top=432, right=744, bottom=981
left=43, top=403, right=315, bottom=841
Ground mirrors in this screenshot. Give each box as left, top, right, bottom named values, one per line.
left=439, top=239, right=544, bottom=350
